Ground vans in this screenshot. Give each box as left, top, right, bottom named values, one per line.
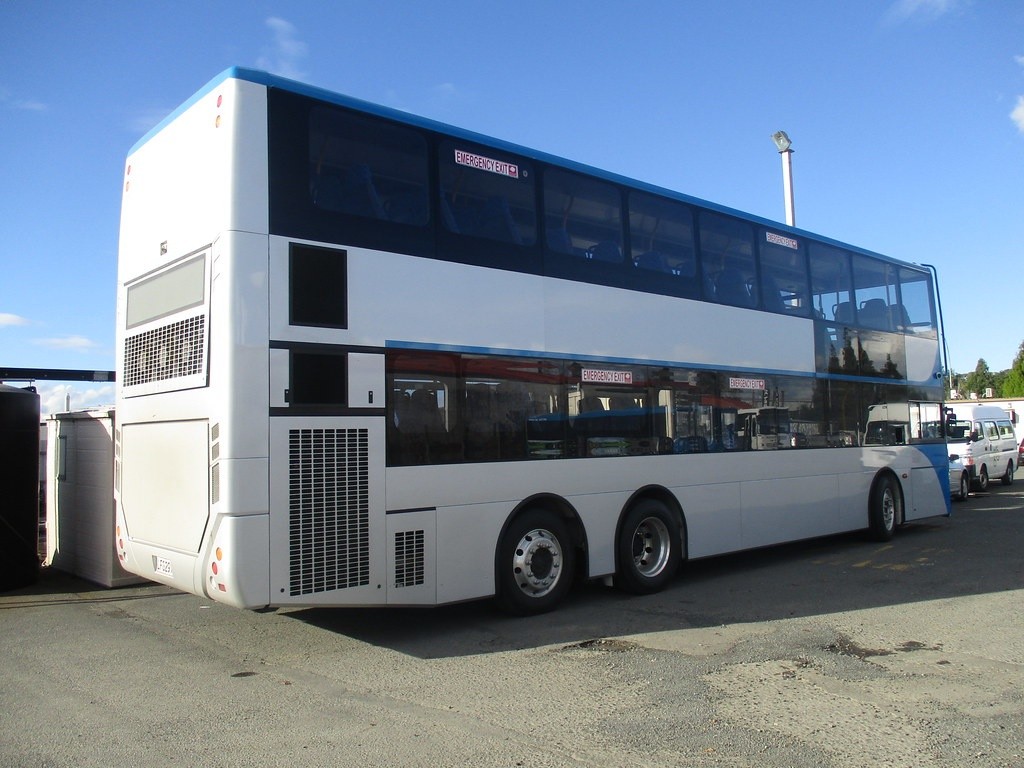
left=862, top=402, right=940, bottom=446
left=945, top=404, right=1020, bottom=491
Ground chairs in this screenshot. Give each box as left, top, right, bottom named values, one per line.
left=310, top=155, right=919, bottom=467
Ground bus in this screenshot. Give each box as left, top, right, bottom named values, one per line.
left=827, top=431, right=857, bottom=447
left=734, top=406, right=792, bottom=450
left=111, top=65, right=953, bottom=614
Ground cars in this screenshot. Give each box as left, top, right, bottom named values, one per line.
left=948, top=453, right=969, bottom=500
left=790, top=432, right=808, bottom=448
left=1018, top=437, right=1024, bottom=466
